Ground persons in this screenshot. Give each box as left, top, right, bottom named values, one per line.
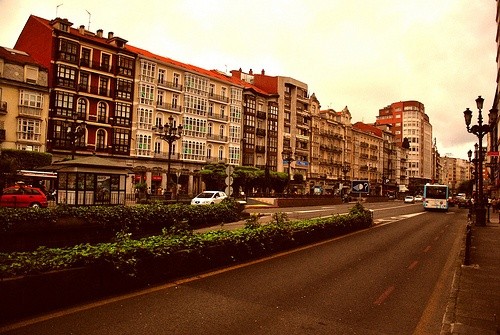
left=42, top=181, right=46, bottom=189
left=341, top=194, right=345, bottom=205
left=457, top=198, right=460, bottom=207
left=146, top=187, right=152, bottom=201
left=135, top=189, right=140, bottom=203
left=488, top=197, right=492, bottom=207
left=471, top=197, right=475, bottom=206
left=491, top=196, right=496, bottom=213
left=497, top=198, right=500, bottom=213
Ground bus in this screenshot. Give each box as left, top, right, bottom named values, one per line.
left=422, top=183, right=449, bottom=210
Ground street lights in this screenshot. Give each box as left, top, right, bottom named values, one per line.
left=160, top=115, right=183, bottom=192
left=467, top=143, right=486, bottom=207
left=63, top=113, right=87, bottom=159
left=340, top=161, right=351, bottom=193
left=281, top=146, right=298, bottom=193
left=464, top=96, right=499, bottom=224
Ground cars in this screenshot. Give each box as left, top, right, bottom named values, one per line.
left=0, top=186, right=47, bottom=211
left=191, top=191, right=228, bottom=209
left=449, top=192, right=469, bottom=209
left=415, top=195, right=423, bottom=202
left=405, top=196, right=414, bottom=203
